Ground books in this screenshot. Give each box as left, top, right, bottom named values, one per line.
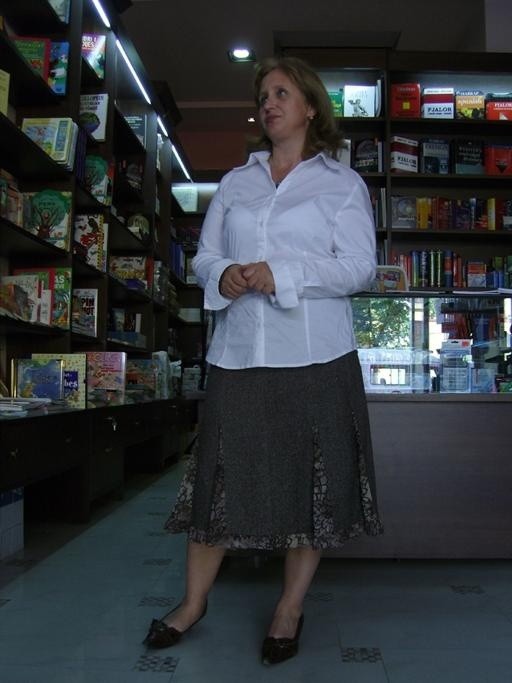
left=73, top=212, right=104, bottom=272
left=8, top=348, right=153, bottom=400
left=0, top=265, right=98, bottom=338
left=21, top=190, right=72, bottom=253
left=378, top=239, right=512, bottom=289
left=21, top=116, right=80, bottom=172
left=9, top=34, right=51, bottom=85
left=79, top=88, right=110, bottom=142
left=47, top=39, right=71, bottom=97
left=370, top=187, right=501, bottom=231
left=123, top=112, right=147, bottom=148
left=81, top=31, right=107, bottom=80
left=323, top=79, right=512, bottom=175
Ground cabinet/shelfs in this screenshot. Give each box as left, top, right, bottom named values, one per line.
left=1, top=1, right=197, bottom=583
left=172, top=169, right=228, bottom=392
left=274, top=31, right=508, bottom=560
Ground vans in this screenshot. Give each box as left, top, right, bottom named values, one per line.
left=357, top=349, right=440, bottom=394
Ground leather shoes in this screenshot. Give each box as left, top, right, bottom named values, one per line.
left=261, top=613, right=305, bottom=665
left=144, top=598, right=208, bottom=649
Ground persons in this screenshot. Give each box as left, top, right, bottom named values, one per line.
left=141, top=55, right=382, bottom=665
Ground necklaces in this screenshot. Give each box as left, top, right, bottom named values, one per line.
left=270, top=160, right=299, bottom=188
